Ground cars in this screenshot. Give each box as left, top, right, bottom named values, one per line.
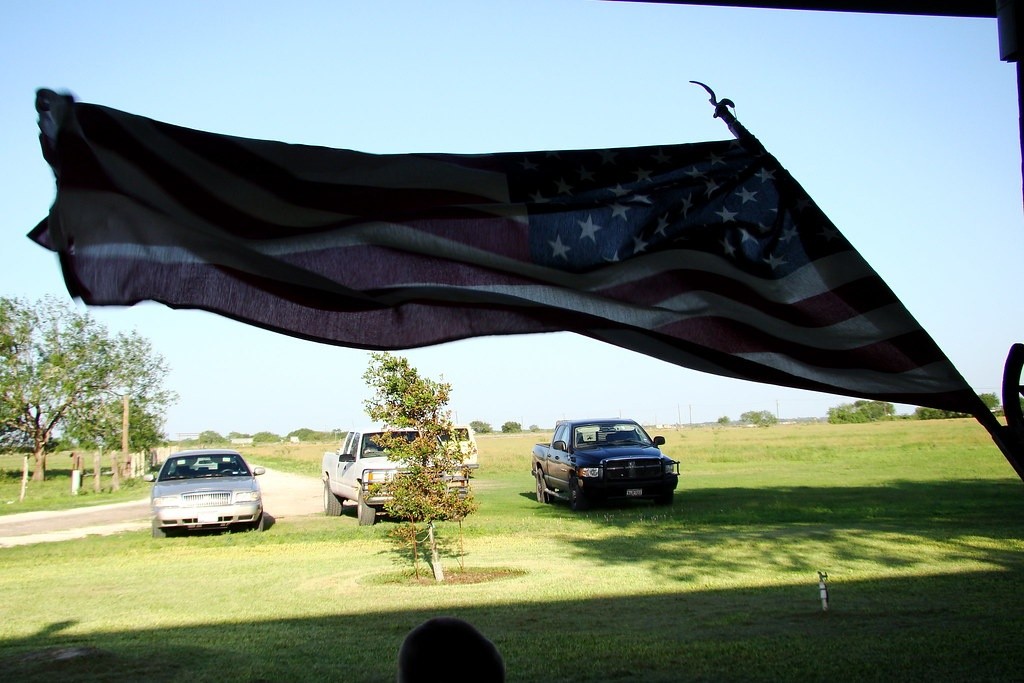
left=142, top=449, right=266, bottom=540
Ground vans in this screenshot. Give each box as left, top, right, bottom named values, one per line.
left=432, top=423, right=480, bottom=479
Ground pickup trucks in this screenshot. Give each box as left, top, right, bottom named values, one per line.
left=530, top=417, right=680, bottom=513
left=321, top=421, right=469, bottom=527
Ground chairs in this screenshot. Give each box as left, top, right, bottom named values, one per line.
left=173, top=460, right=237, bottom=477
left=580, top=428, right=639, bottom=441
